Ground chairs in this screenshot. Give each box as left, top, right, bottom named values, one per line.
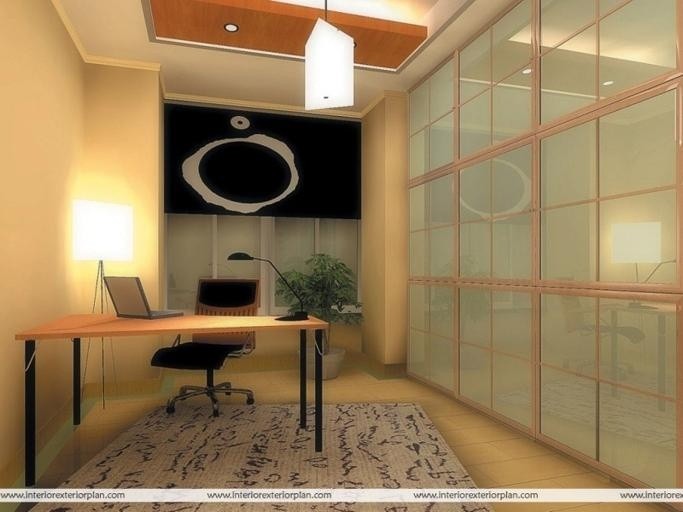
left=151, top=275, right=259, bottom=417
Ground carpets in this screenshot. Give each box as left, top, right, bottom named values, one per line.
left=30, top=399, right=488, bottom=512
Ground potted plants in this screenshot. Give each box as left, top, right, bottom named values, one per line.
left=273, top=253, right=363, bottom=380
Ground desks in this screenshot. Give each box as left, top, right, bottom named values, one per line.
left=14, top=313, right=329, bottom=489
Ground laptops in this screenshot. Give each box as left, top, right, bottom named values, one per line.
left=103, top=276, right=184, bottom=319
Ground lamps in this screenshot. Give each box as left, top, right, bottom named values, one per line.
left=227, top=252, right=309, bottom=321
left=304, top=0, right=356, bottom=109
